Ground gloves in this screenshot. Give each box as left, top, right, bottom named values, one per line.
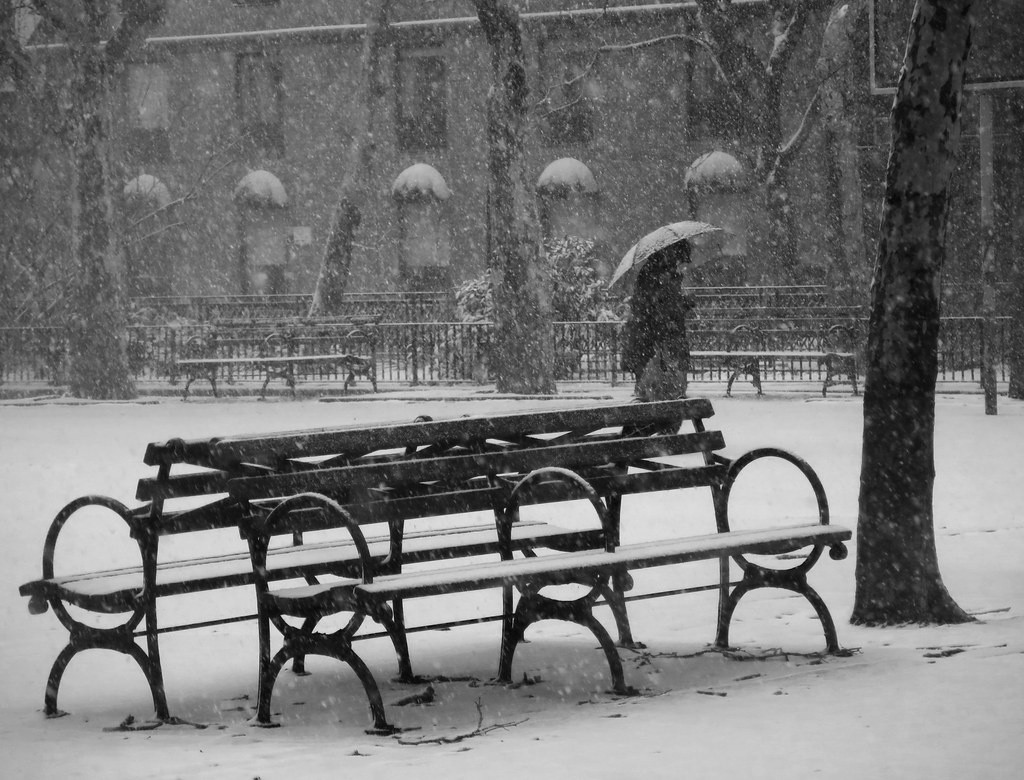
left=669, top=272, right=684, bottom=292
left=683, top=293, right=695, bottom=310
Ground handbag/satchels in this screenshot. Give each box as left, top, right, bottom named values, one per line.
left=634, top=347, right=689, bottom=402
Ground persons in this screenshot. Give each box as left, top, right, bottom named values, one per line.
left=623, top=240, right=697, bottom=434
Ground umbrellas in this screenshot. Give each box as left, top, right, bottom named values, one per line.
left=606, top=220, right=735, bottom=296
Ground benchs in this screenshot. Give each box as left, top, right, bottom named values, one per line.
left=218, top=395, right=854, bottom=736
left=173, top=329, right=377, bottom=401
left=685, top=324, right=861, bottom=400
left=18, top=412, right=634, bottom=737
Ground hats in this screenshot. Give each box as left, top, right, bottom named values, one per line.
left=667, top=239, right=692, bottom=263
